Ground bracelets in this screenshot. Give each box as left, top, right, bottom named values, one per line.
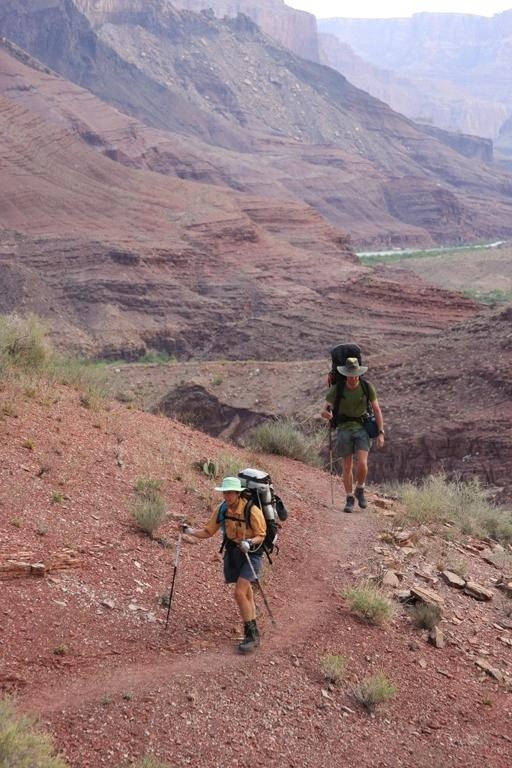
left=246, top=539, right=255, bottom=551
left=377, top=429, right=384, bottom=434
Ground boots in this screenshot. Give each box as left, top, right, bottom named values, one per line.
left=239, top=619, right=260, bottom=653
left=344, top=487, right=367, bottom=513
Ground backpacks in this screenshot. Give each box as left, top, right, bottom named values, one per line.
left=218, top=468, right=288, bottom=551
left=328, top=343, right=372, bottom=430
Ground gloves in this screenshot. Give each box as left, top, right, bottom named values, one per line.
left=239, top=540, right=251, bottom=553
left=180, top=523, right=190, bottom=533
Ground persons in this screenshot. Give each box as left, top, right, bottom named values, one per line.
left=179, top=477, right=267, bottom=652
left=320, top=357, right=385, bottom=513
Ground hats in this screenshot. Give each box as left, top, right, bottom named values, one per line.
left=215, top=477, right=247, bottom=492
left=337, top=357, right=368, bottom=376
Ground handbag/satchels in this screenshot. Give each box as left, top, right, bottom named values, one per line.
left=365, top=417, right=377, bottom=439
